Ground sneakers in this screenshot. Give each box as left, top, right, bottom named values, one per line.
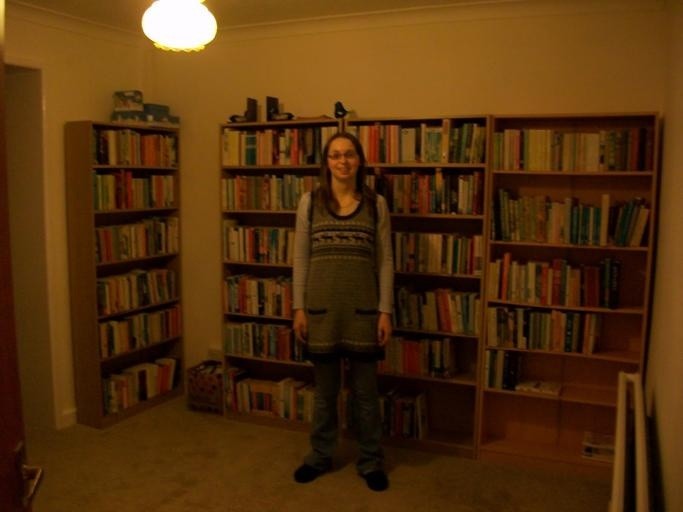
left=359, top=467, right=392, bottom=493
left=293, top=458, right=334, bottom=484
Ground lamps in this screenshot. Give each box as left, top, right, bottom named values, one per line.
left=140, top=0, right=218, bottom=54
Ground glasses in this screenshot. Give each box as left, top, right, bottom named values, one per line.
left=327, top=151, right=360, bottom=161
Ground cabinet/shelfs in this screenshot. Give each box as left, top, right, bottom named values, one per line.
left=212, top=117, right=341, bottom=432
left=335, top=114, right=484, bottom=468
left=60, top=117, right=188, bottom=430
left=475, top=107, right=663, bottom=484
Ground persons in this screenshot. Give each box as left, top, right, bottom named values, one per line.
left=290, top=134, right=394, bottom=493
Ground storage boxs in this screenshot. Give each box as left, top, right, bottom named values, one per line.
left=112, top=88, right=145, bottom=114
left=580, top=428, right=617, bottom=463
left=186, top=362, right=224, bottom=416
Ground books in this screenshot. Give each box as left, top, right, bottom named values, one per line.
left=94, top=124, right=183, bottom=420
